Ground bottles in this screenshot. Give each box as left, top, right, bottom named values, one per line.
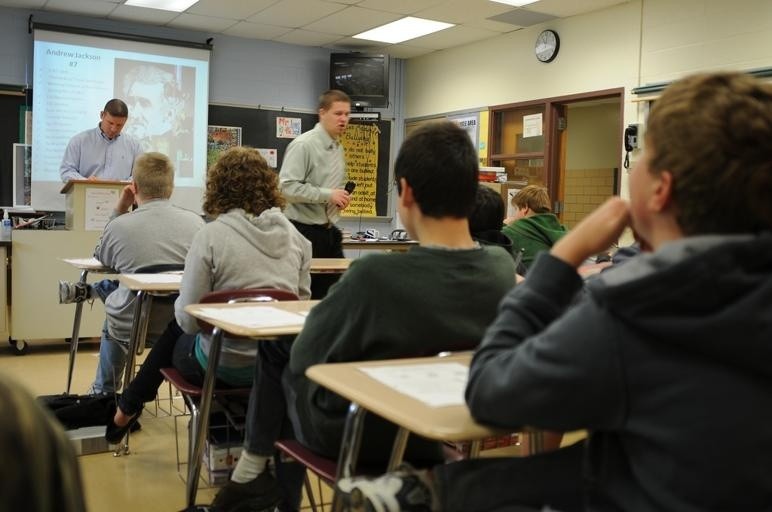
left=2, top=208, right=11, bottom=241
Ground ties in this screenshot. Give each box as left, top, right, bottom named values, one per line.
left=327, top=142, right=340, bottom=223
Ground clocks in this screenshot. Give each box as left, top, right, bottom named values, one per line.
left=535, top=30, right=560, bottom=63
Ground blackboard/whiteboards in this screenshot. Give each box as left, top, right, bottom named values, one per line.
left=209, top=102, right=394, bottom=223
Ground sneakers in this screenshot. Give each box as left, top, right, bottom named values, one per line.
left=106, top=408, right=142, bottom=444
left=59, top=281, right=91, bottom=303
left=212, top=459, right=282, bottom=512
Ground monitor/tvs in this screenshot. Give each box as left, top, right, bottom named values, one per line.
left=329, top=52, right=389, bottom=113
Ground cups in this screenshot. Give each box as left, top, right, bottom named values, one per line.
left=366, top=229, right=379, bottom=242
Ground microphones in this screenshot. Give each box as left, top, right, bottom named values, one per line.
left=337, top=181, right=356, bottom=205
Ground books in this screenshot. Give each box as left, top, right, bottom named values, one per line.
left=476, top=167, right=508, bottom=182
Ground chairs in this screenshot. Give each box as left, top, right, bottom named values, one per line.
left=160, top=288, right=299, bottom=487
left=102, top=265, right=185, bottom=416
left=275, top=352, right=481, bottom=511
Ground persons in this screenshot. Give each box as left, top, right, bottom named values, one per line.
left=84, top=152, right=205, bottom=394
left=104, top=144, right=313, bottom=444
left=0, top=368, right=87, bottom=512
left=501, top=183, right=567, bottom=270
left=58, top=98, right=144, bottom=185
left=205, top=118, right=516, bottom=512
left=467, top=182, right=528, bottom=275
left=428, top=70, right=772, bottom=510
left=121, top=64, right=192, bottom=176
left=276, top=90, right=358, bottom=300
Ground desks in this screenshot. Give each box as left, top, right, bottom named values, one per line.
left=61, top=257, right=371, bottom=395
left=184, top=299, right=337, bottom=511
left=342, top=237, right=418, bottom=252
left=304, top=350, right=565, bottom=511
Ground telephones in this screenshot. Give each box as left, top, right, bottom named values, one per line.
left=625, top=128, right=634, bottom=151
